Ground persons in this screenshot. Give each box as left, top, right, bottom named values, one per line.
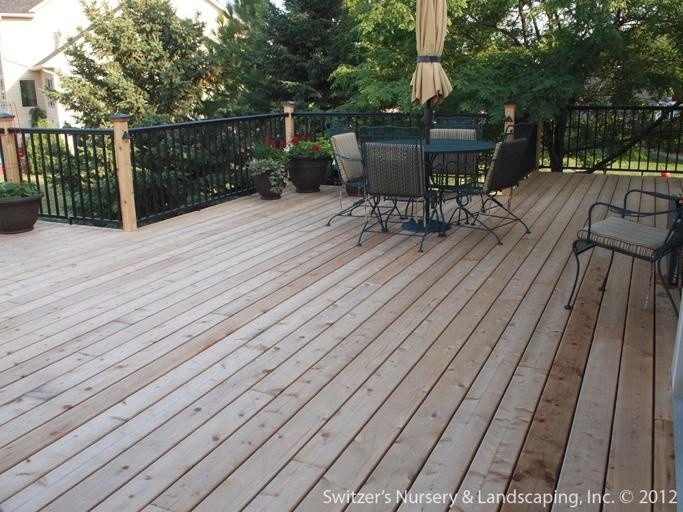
left=17, top=139, right=32, bottom=173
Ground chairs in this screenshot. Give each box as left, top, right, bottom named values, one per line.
left=324, top=114, right=539, bottom=252
left=564, top=189, right=683, bottom=320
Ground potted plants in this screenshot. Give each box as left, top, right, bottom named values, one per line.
left=0, top=180, right=44, bottom=234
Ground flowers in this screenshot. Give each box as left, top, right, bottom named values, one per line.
left=246, top=128, right=290, bottom=197
left=286, top=136, right=334, bottom=159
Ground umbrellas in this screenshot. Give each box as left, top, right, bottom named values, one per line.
left=398, top=0, right=451, bottom=232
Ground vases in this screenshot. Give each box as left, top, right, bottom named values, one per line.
left=253, top=167, right=287, bottom=199
left=286, top=156, right=329, bottom=192
